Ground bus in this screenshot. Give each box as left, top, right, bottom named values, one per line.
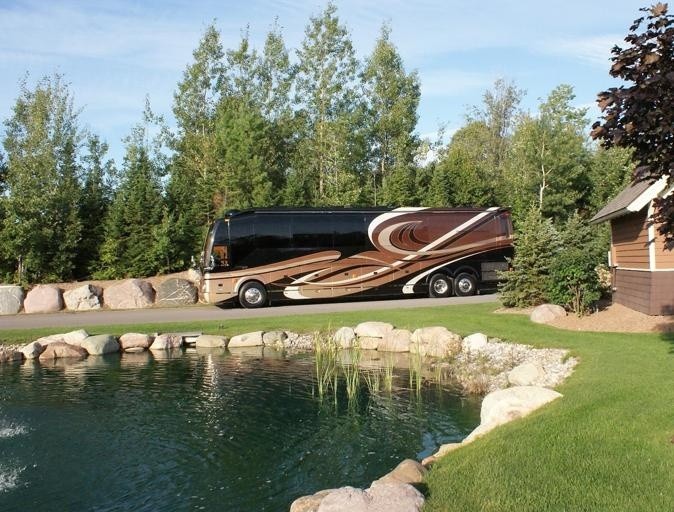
left=190, top=207, right=516, bottom=309
